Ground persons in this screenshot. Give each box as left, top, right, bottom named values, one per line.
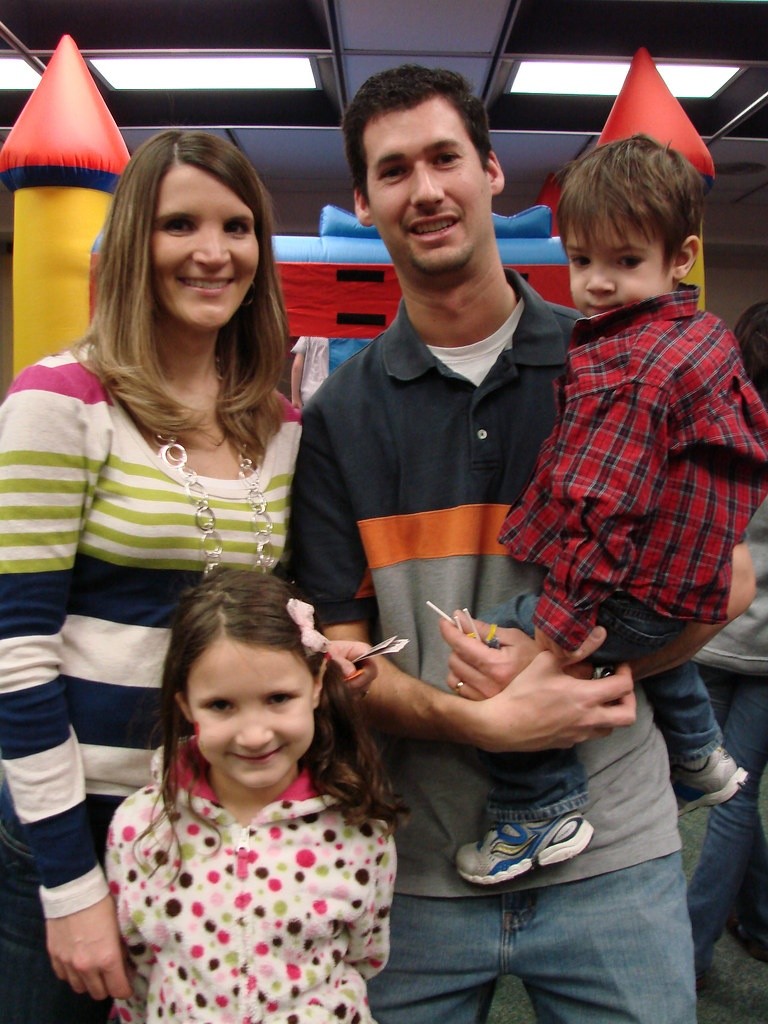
left=289, top=336, right=330, bottom=410
left=2, top=124, right=305, bottom=1024
left=452, top=137, right=768, bottom=886
left=101, top=566, right=402, bottom=1024
left=685, top=303, right=767, bottom=986
left=271, top=62, right=760, bottom=1024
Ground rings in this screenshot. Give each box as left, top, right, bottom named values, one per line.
left=485, top=623, right=497, bottom=643
left=455, top=680, right=465, bottom=693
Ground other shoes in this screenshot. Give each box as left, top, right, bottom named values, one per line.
left=726, top=912, right=768, bottom=962
left=696, top=975, right=705, bottom=990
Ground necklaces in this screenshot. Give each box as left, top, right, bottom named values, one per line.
left=155, top=429, right=277, bottom=579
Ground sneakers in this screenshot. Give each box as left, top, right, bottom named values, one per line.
left=457, top=809, right=594, bottom=884
left=670, top=747, right=750, bottom=816
